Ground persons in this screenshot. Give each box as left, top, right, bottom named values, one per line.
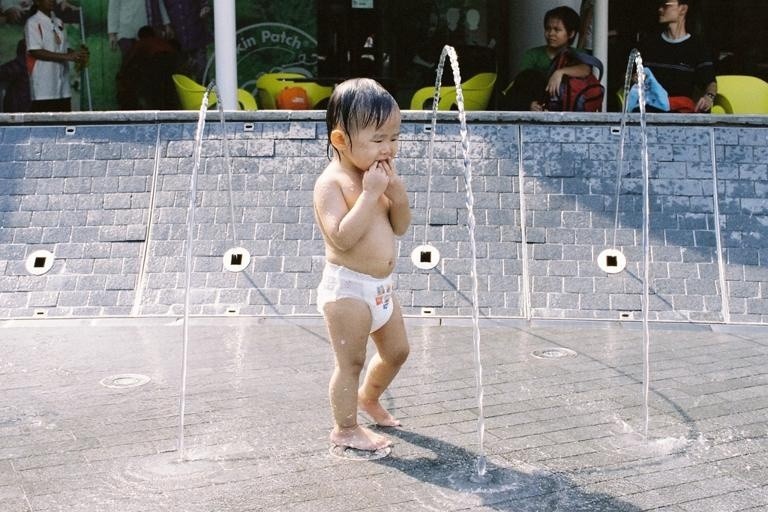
left=503, top=4, right=593, bottom=112
left=307, top=76, right=417, bottom=453
left=116, top=24, right=191, bottom=110
left=615, top=0, right=718, bottom=113
left=106, top=0, right=175, bottom=67
left=23, top=0, right=90, bottom=111
left=3, top=67, right=33, bottom=112
left=0, top=38, right=27, bottom=112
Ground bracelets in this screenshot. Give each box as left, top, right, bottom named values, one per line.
left=704, top=91, right=716, bottom=101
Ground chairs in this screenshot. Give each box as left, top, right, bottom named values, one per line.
left=256, top=71, right=333, bottom=109
left=710, top=75, right=767, bottom=113
left=410, top=72, right=494, bottom=110
left=172, top=74, right=257, bottom=112
left=616, top=90, right=734, bottom=114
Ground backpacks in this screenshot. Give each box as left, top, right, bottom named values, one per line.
left=541, top=45, right=605, bottom=112
